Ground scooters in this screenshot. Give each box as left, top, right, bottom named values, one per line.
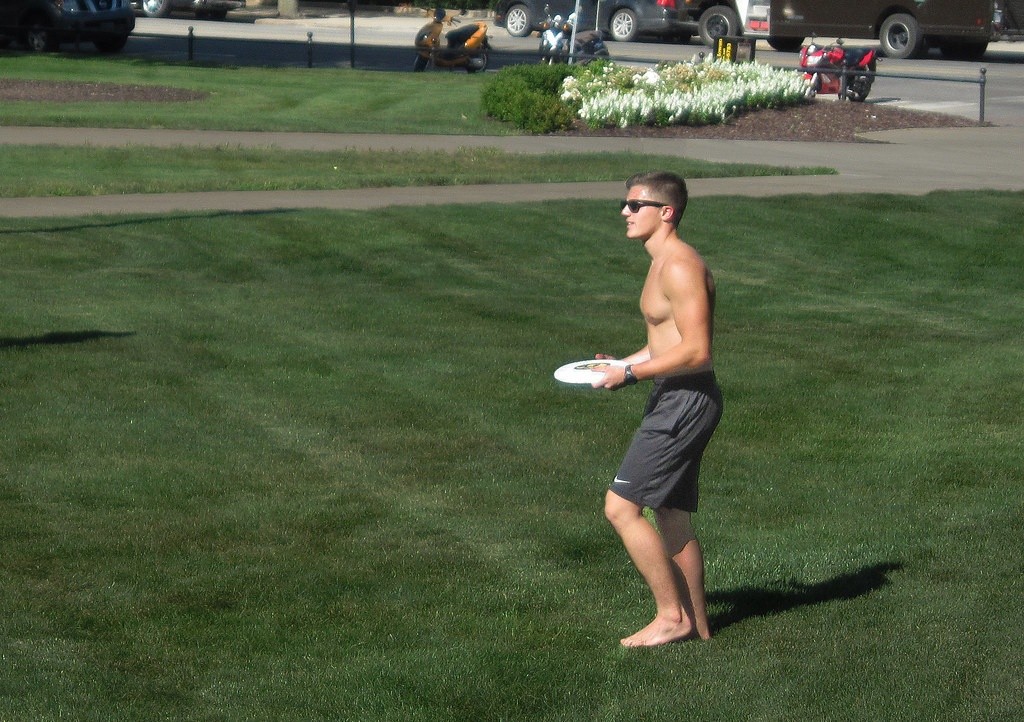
left=414, top=0, right=494, bottom=74
left=539, top=5, right=609, bottom=65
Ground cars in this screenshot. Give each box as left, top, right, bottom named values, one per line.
left=688, top=1, right=1004, bottom=59
left=493, top=0, right=699, bottom=42
left=0, top=0, right=136, bottom=54
left=132, top=0, right=247, bottom=19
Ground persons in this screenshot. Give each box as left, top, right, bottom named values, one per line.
left=591, top=170, right=722, bottom=647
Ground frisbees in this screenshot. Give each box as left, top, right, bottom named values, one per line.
left=553, top=358, right=639, bottom=385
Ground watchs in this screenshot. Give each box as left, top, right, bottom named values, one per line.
left=625, top=365, right=636, bottom=388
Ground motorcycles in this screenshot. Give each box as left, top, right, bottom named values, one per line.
left=798, top=32, right=884, bottom=102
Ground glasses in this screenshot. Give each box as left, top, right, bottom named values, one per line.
left=619, top=197, right=668, bottom=214
